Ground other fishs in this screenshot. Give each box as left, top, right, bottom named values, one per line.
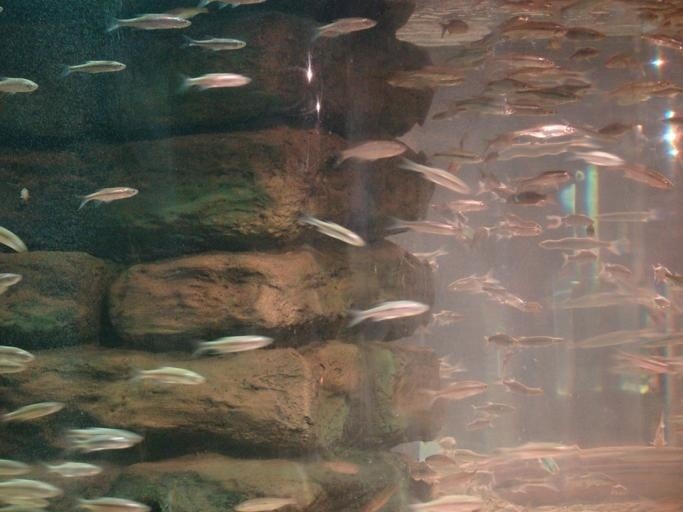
left=295, top=0, right=683, bottom=512
